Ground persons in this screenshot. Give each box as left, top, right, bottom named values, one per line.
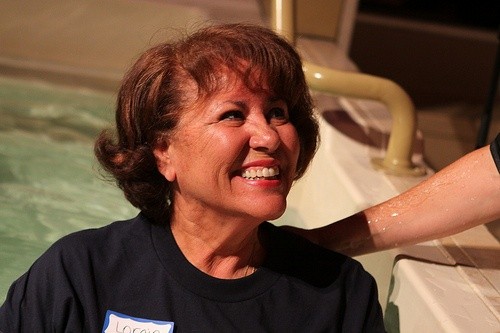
left=278, top=129, right=500, bottom=257
left=0, top=20, right=386, bottom=333
